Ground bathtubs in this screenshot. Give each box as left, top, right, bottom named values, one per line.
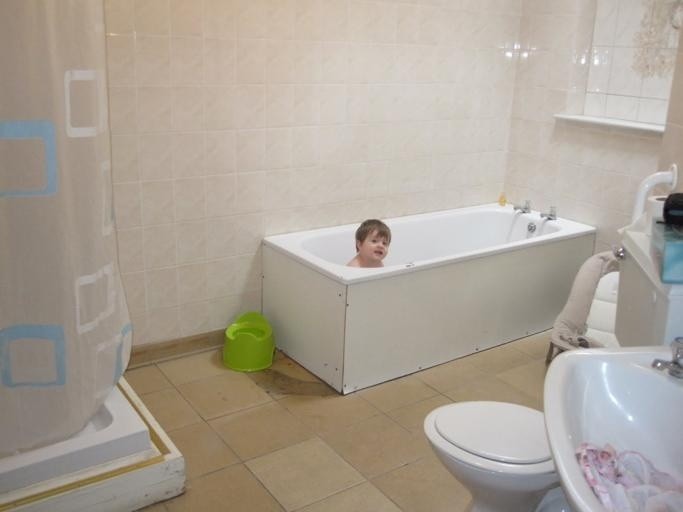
left=260, top=201, right=596, bottom=396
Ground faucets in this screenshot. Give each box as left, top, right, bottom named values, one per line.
left=650, top=337, right=683, bottom=380
left=539, top=206, right=556, bottom=220
left=513, top=200, right=530, bottom=214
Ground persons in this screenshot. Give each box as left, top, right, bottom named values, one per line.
left=347, top=217, right=393, bottom=269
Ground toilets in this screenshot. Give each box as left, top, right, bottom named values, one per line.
left=423, top=334, right=602, bottom=512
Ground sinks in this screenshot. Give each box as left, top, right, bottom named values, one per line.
left=543, top=346, right=683, bottom=512
